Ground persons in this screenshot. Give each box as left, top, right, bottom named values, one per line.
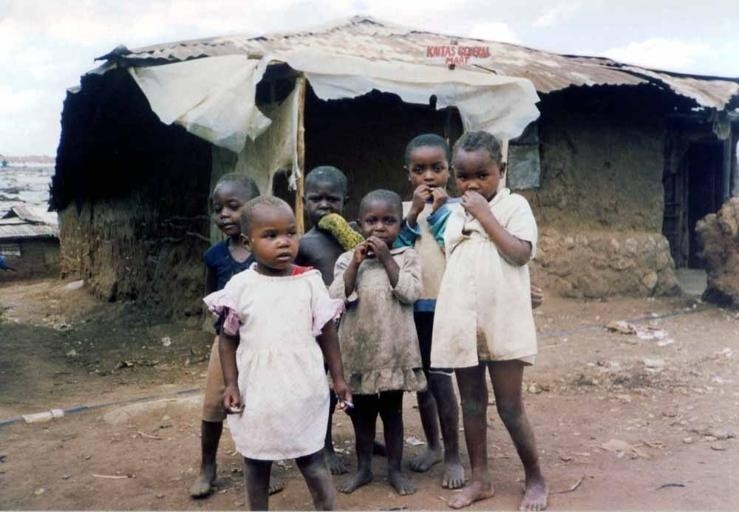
left=428, top=131, right=550, bottom=511
left=328, top=188, right=429, bottom=494
left=204, top=194, right=353, bottom=511
left=291, top=165, right=389, bottom=476
left=188, top=171, right=288, bottom=497
left=392, top=134, right=466, bottom=490
